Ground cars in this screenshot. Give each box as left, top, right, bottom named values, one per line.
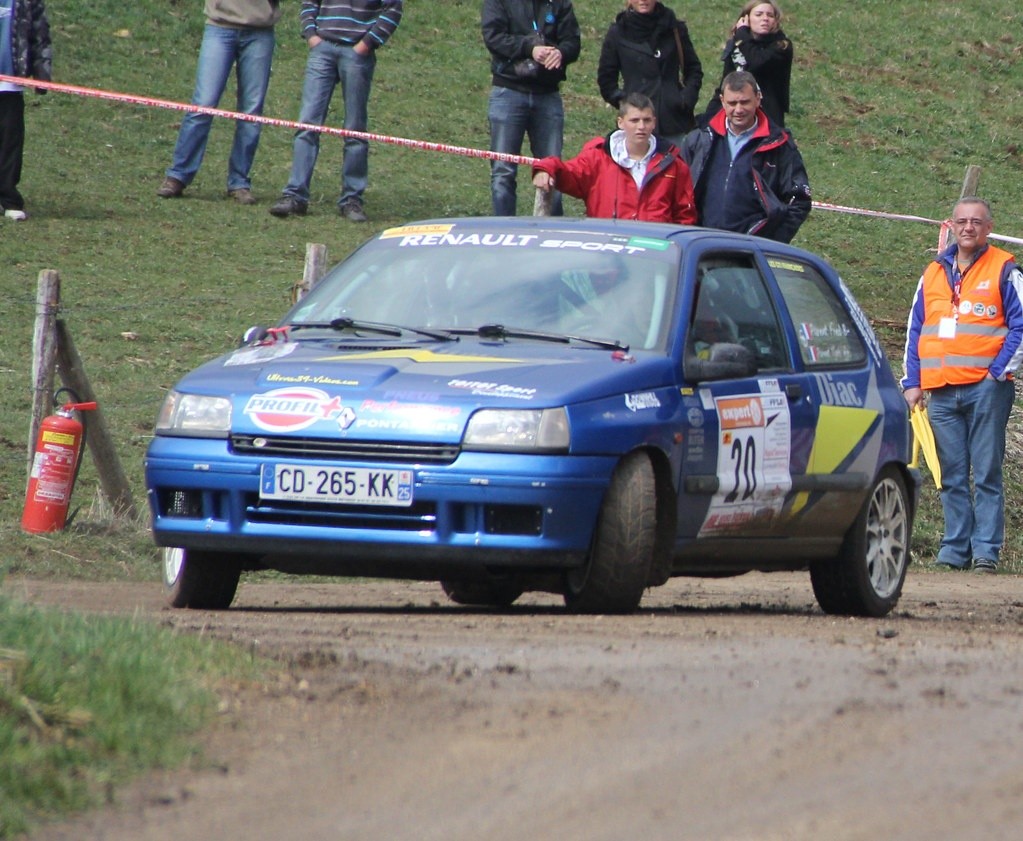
left=144, top=214, right=922, bottom=618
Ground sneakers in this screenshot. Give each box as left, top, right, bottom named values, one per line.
left=159, top=175, right=187, bottom=197
left=338, top=198, right=368, bottom=222
left=268, top=197, right=307, bottom=216
left=229, top=188, right=258, bottom=206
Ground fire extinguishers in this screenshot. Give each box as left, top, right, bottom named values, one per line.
left=22, top=388, right=95, bottom=531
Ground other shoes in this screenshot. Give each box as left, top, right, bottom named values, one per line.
left=6, top=209, right=27, bottom=221
left=931, top=559, right=1003, bottom=572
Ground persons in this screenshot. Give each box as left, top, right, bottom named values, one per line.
left=530, top=93, right=697, bottom=225
left=900, top=196, right=1023, bottom=573
left=704, top=0, right=794, bottom=128
left=482, top=0, right=581, bottom=216
left=269, top=0, right=402, bottom=222
left=596, top=0, right=704, bottom=136
left=680, top=71, right=811, bottom=244
left=156, top=0, right=281, bottom=205
left=0, top=0, right=52, bottom=220
